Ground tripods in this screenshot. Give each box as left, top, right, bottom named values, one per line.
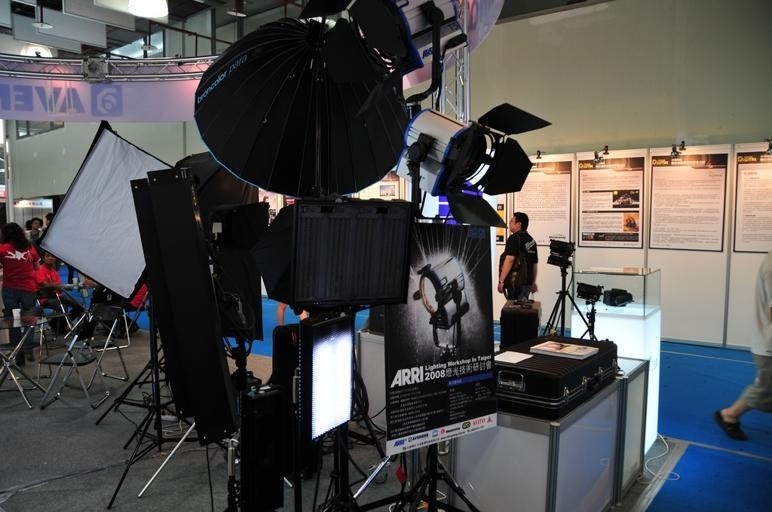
left=392, top=443, right=480, bottom=512
left=543, top=267, right=598, bottom=341
left=94, top=297, right=228, bottom=510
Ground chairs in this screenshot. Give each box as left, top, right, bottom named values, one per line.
left=0, top=293, right=132, bottom=412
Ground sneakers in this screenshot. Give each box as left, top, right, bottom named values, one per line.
left=26, top=353, right=36, bottom=362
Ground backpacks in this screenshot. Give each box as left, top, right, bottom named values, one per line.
left=497, top=247, right=530, bottom=301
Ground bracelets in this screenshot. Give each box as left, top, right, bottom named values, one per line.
left=498, top=280, right=504, bottom=284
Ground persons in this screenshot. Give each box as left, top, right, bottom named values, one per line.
left=274, top=301, right=310, bottom=326
left=712, top=246, right=771, bottom=443
left=24, top=210, right=150, bottom=338
left=497, top=209, right=540, bottom=305
left=0, top=222, right=41, bottom=367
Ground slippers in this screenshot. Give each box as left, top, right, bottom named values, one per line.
left=711, top=408, right=750, bottom=441
